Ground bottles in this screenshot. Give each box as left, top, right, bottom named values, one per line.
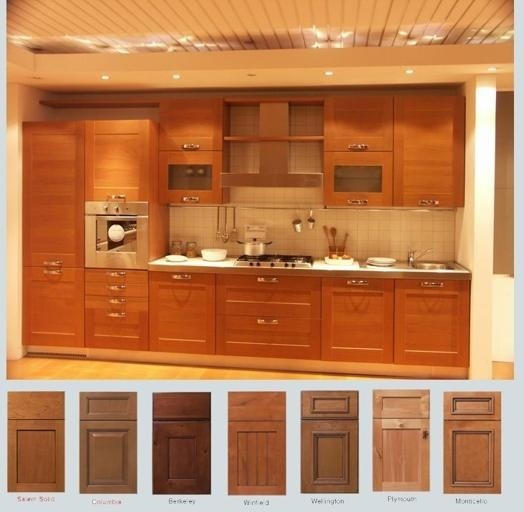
left=185, top=241, right=196, bottom=257
left=170, top=241, right=181, bottom=256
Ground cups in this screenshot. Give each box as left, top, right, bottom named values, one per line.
left=324, top=246, right=353, bottom=266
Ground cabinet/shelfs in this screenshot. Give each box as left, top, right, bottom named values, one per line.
left=393, top=94, right=465, bottom=209
left=146, top=272, right=214, bottom=367
left=443, top=391, right=501, bottom=496
left=227, top=390, right=286, bottom=495
left=83, top=268, right=148, bottom=364
left=372, top=387, right=429, bottom=494
left=21, top=264, right=85, bottom=359
left=320, top=97, right=393, bottom=208
left=214, top=274, right=320, bottom=374
left=7, top=391, right=64, bottom=493
left=300, top=390, right=360, bottom=494
left=85, top=119, right=169, bottom=204
left=153, top=392, right=211, bottom=496
left=156, top=98, right=231, bottom=207
left=78, top=391, right=137, bottom=494
left=20, top=120, right=86, bottom=267
left=321, top=279, right=471, bottom=380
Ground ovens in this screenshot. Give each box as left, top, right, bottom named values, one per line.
left=83, top=214, right=146, bottom=270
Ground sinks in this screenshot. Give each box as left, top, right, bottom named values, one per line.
left=411, top=261, right=456, bottom=271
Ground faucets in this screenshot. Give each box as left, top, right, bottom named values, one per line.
left=407, top=247, right=434, bottom=268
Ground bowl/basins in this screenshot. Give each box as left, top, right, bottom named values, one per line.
left=200, top=248, right=226, bottom=261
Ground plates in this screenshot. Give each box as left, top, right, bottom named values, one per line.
left=165, top=255, right=186, bottom=262
left=366, top=257, right=397, bottom=266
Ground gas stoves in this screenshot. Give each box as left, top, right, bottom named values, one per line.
left=235, top=254, right=314, bottom=268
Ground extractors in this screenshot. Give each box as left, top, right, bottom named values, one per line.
left=218, top=103, right=323, bottom=188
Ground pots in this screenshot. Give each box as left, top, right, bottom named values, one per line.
left=236, top=238, right=272, bottom=256
